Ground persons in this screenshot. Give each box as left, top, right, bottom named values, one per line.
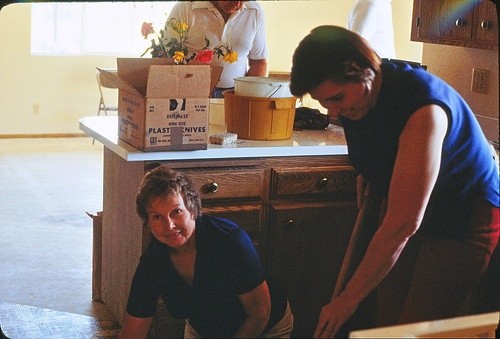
left=156, top=1, right=269, bottom=99
left=290, top=25, right=500, bottom=339
left=117, top=164, right=294, bottom=339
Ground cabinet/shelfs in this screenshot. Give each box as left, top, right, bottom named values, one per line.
left=410, top=0, right=499, bottom=51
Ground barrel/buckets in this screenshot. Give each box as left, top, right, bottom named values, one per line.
left=209, top=97, right=225, bottom=126
left=233, top=75, right=294, bottom=98
left=221, top=89, right=297, bottom=141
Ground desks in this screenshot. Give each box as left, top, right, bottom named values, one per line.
left=79, top=116, right=357, bottom=326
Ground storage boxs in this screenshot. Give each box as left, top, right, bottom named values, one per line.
left=96, top=58, right=223, bottom=153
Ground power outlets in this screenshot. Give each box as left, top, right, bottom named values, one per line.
left=471, top=68, right=489, bottom=94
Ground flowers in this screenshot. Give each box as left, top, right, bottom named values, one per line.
left=140, top=17, right=238, bottom=67
